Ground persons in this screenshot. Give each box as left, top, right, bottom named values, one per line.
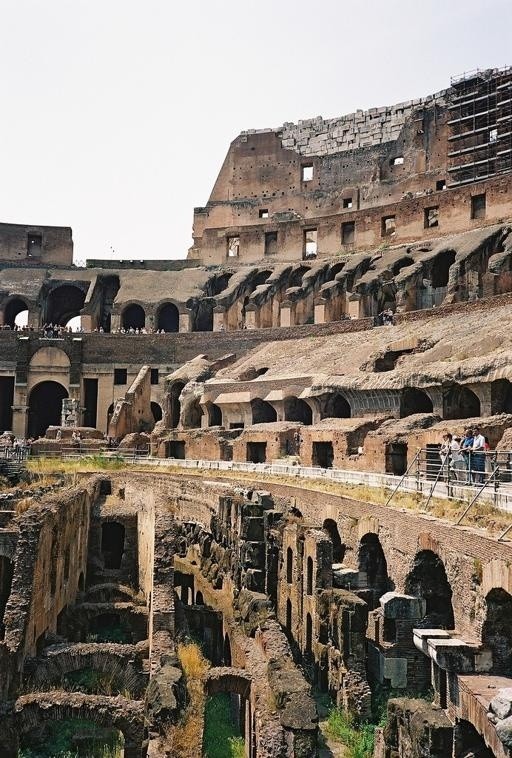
left=340, top=308, right=393, bottom=326
left=238, top=321, right=243, bottom=330
left=217, top=321, right=223, bottom=331
left=1, top=322, right=166, bottom=340
left=293, top=430, right=303, bottom=455
left=439, top=428, right=492, bottom=487
left=242, top=322, right=247, bottom=329
left=0, top=427, right=85, bottom=461
